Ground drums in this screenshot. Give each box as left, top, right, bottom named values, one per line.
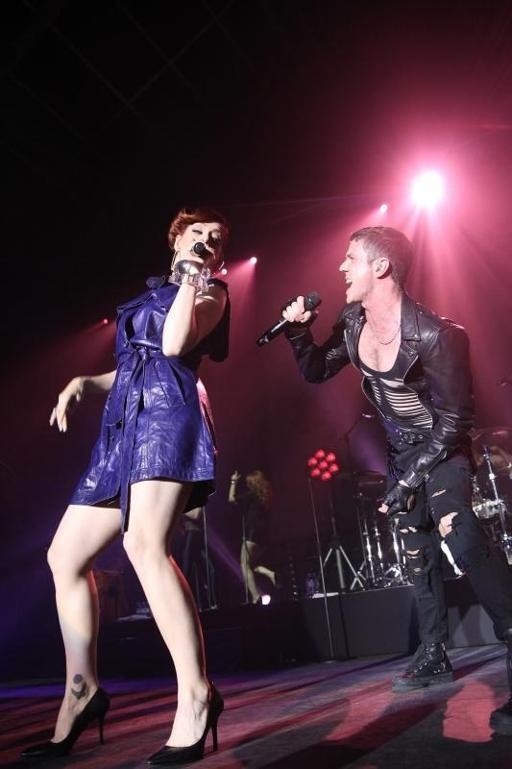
left=472, top=498, right=507, bottom=524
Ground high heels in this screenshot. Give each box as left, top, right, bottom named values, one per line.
left=20, top=688, right=110, bottom=760
left=145, top=681, right=223, bottom=765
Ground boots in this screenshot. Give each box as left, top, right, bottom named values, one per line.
left=489, top=629, right=512, bottom=735
left=392, top=641, right=452, bottom=688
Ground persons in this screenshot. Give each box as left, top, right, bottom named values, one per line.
left=21, top=193, right=233, bottom=766
left=226, top=467, right=279, bottom=604
left=280, top=223, right=512, bottom=726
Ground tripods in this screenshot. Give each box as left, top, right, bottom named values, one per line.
left=315, top=416, right=415, bottom=591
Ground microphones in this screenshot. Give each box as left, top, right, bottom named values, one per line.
left=193, top=242, right=204, bottom=253
left=256, top=294, right=321, bottom=346
